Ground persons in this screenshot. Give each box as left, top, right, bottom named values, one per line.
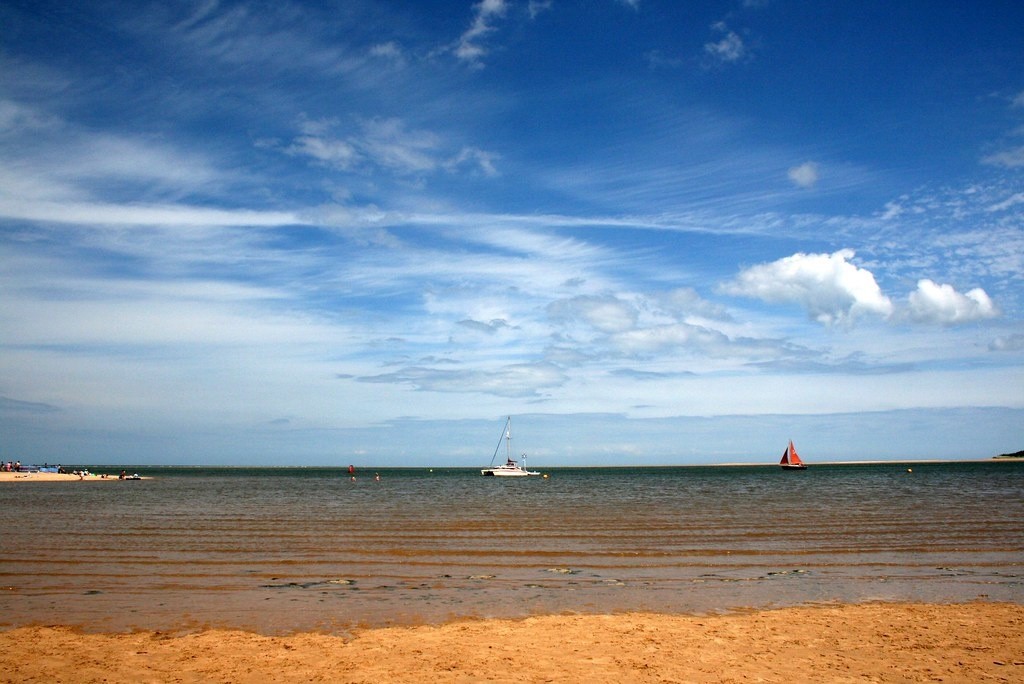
left=0, top=461, right=139, bottom=480
left=352, top=472, right=380, bottom=482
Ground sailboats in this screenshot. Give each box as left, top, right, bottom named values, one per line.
left=778, top=439, right=808, bottom=470
left=480, top=416, right=541, bottom=477
